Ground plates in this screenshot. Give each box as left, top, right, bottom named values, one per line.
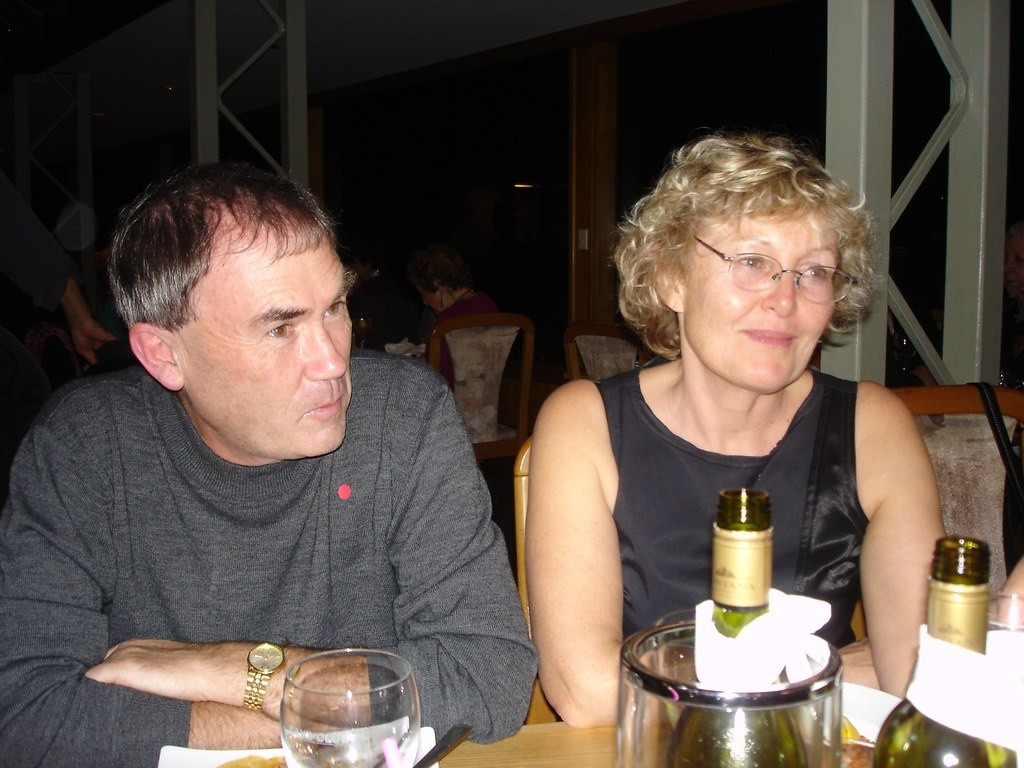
left=157, top=727, right=441, bottom=768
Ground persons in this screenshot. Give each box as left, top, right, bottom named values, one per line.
left=523, top=133, right=1024, bottom=729
left=1, top=164, right=541, bottom=768
left=1, top=169, right=501, bottom=515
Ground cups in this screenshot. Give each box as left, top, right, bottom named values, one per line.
left=987, top=593, right=1024, bottom=631
left=613, top=608, right=843, bottom=768
left=280, top=647, right=420, bottom=768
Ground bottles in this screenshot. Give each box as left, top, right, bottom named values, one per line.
left=870, top=536, right=1017, bottom=768
left=665, top=487, right=807, bottom=768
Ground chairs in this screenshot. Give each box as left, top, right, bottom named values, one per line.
left=890, top=383, right=1024, bottom=622
left=428, top=313, right=651, bottom=725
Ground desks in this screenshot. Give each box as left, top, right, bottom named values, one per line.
left=435, top=711, right=876, bottom=768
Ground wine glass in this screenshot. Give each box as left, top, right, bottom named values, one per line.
left=354, top=312, right=374, bottom=350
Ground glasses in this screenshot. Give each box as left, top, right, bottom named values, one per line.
left=694, top=235, right=857, bottom=305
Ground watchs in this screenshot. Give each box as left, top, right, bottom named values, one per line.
left=242, top=637, right=304, bottom=710
